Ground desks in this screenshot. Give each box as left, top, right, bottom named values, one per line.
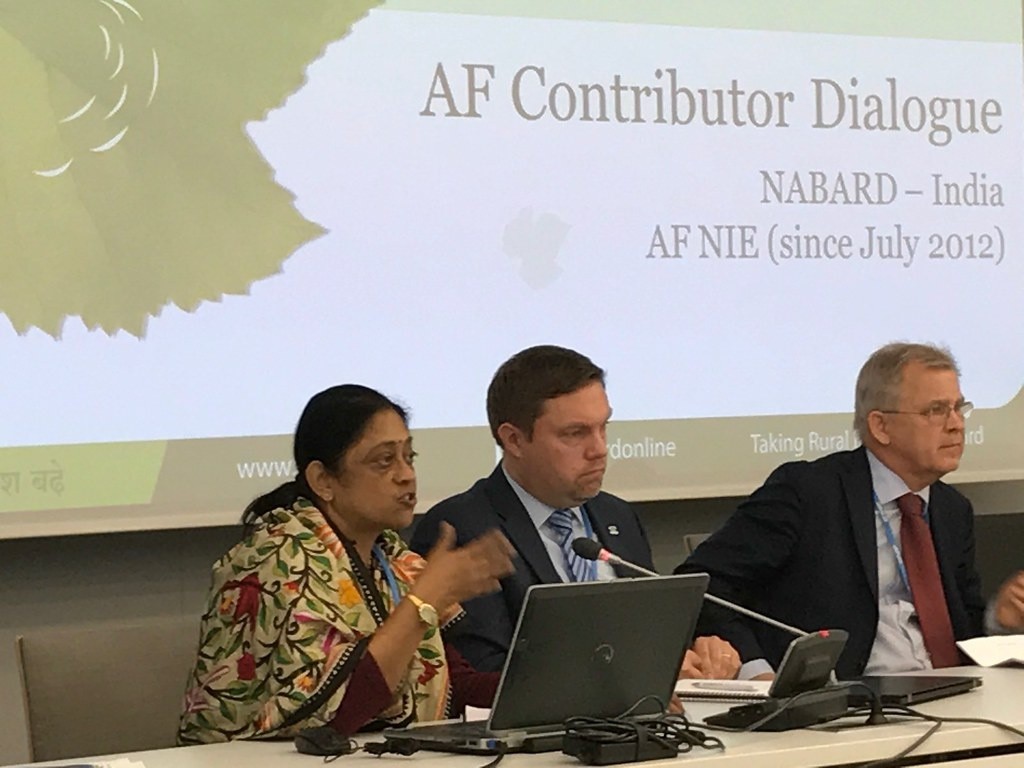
left=0, top=665, right=1024, bottom=768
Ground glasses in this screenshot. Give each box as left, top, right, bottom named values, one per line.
left=874, top=400, right=974, bottom=424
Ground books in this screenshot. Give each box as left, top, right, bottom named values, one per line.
left=669, top=676, right=778, bottom=704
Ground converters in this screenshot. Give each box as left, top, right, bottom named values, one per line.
left=562, top=732, right=679, bottom=766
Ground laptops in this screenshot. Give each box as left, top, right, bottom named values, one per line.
left=837, top=675, right=983, bottom=707
left=383, top=573, right=709, bottom=752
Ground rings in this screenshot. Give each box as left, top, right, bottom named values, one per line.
left=721, top=653, right=732, bottom=658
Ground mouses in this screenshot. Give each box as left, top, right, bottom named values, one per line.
left=295, top=726, right=351, bottom=756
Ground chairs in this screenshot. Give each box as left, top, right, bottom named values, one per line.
left=16, top=615, right=204, bottom=762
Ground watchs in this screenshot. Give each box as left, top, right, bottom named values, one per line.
left=407, top=593, right=439, bottom=627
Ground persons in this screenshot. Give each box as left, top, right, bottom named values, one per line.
left=406, top=343, right=745, bottom=720
left=174, top=379, right=520, bottom=748
left=669, top=341, right=1024, bottom=685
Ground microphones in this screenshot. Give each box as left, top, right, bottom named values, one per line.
left=572, top=538, right=850, bottom=732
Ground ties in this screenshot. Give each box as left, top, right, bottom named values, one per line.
left=897, top=492, right=958, bottom=670
left=548, top=510, right=597, bottom=583
